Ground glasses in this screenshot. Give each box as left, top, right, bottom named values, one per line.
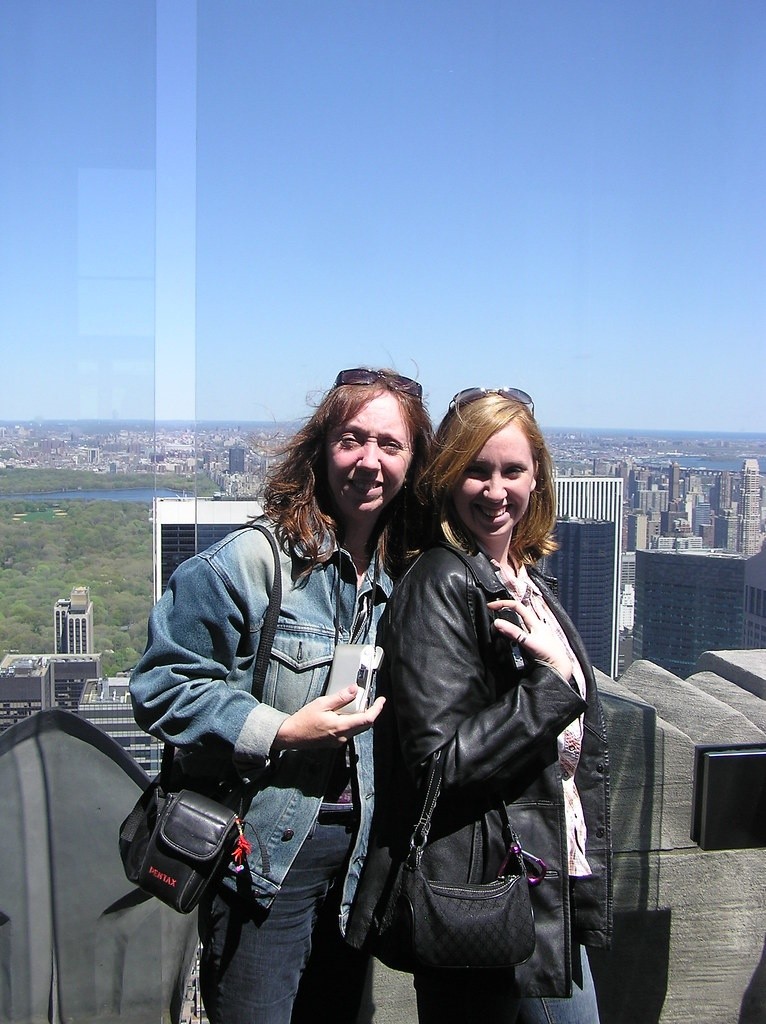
left=327, top=369, right=422, bottom=406
left=449, top=388, right=533, bottom=415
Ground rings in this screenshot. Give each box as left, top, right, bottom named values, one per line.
left=516, top=631, right=528, bottom=644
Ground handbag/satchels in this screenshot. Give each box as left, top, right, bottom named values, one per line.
left=370, top=742, right=536, bottom=971
left=134, top=787, right=242, bottom=912
left=117, top=733, right=247, bottom=886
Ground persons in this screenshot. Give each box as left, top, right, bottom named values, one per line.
left=345, top=388, right=611, bottom=1024
left=129, top=367, right=434, bottom=1023
